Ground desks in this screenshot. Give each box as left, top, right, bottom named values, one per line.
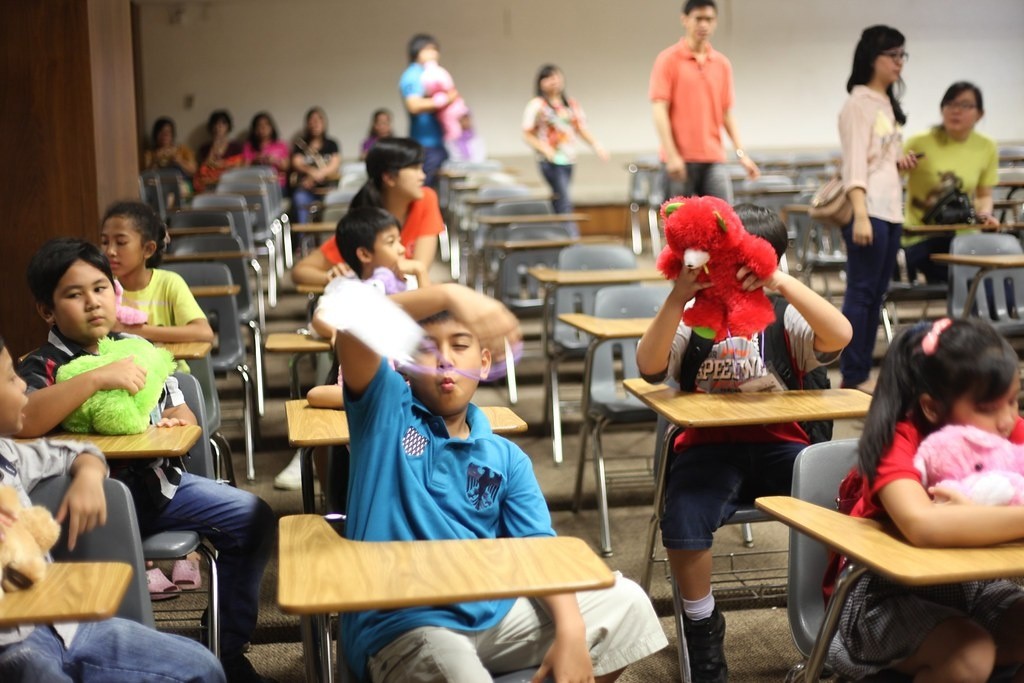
left=151, top=340, right=212, bottom=362
left=276, top=514, right=614, bottom=683
left=529, top=265, right=666, bottom=466
left=624, top=378, right=876, bottom=597
left=38, top=421, right=203, bottom=458
left=261, top=334, right=330, bottom=400
left=750, top=493, right=1024, bottom=683
left=0, top=560, right=134, bottom=623
left=557, top=313, right=654, bottom=557
left=285, top=399, right=529, bottom=515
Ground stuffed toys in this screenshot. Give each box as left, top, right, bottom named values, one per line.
left=915, top=424, right=1024, bottom=508
left=658, top=195, right=778, bottom=342
left=55, top=338, right=177, bottom=436
left=0, top=485, right=61, bottom=600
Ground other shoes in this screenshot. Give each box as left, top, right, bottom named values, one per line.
left=839, top=378, right=876, bottom=394
left=145, top=560, right=202, bottom=600
left=273, top=448, right=306, bottom=491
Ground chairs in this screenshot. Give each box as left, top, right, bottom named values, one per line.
left=0, top=166, right=1023, bottom=683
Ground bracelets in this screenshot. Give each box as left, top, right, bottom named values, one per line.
left=736, top=150, right=746, bottom=158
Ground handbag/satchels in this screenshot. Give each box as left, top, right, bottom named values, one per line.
left=141, top=161, right=193, bottom=205
left=807, top=178, right=852, bottom=226
left=921, top=187, right=971, bottom=225
left=302, top=154, right=338, bottom=194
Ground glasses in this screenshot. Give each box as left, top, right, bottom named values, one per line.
left=946, top=100, right=978, bottom=111
left=879, top=50, right=909, bottom=59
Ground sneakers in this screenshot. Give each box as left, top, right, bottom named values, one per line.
left=681, top=604, right=728, bottom=683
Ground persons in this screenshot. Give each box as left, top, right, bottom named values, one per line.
left=0, top=32, right=473, bottom=683
left=897, top=81, right=1016, bottom=319
left=838, top=25, right=919, bottom=395
left=636, top=204, right=853, bottom=683
left=822, top=315, right=1024, bottom=683
left=522, top=63, right=607, bottom=239
left=335, top=284, right=669, bottom=683
left=646, top=0, right=759, bottom=207
